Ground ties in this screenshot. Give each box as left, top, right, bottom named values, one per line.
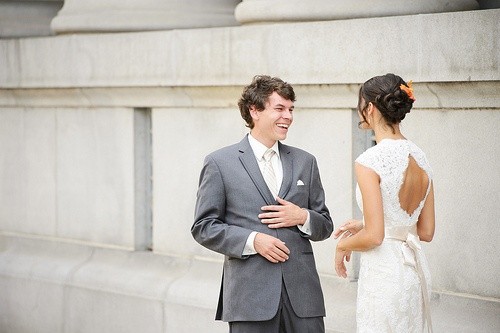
left=263, top=150, right=280, bottom=201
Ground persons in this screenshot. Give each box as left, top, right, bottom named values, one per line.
left=191, top=73, right=335, bottom=333
left=333, top=72, right=436, bottom=333
